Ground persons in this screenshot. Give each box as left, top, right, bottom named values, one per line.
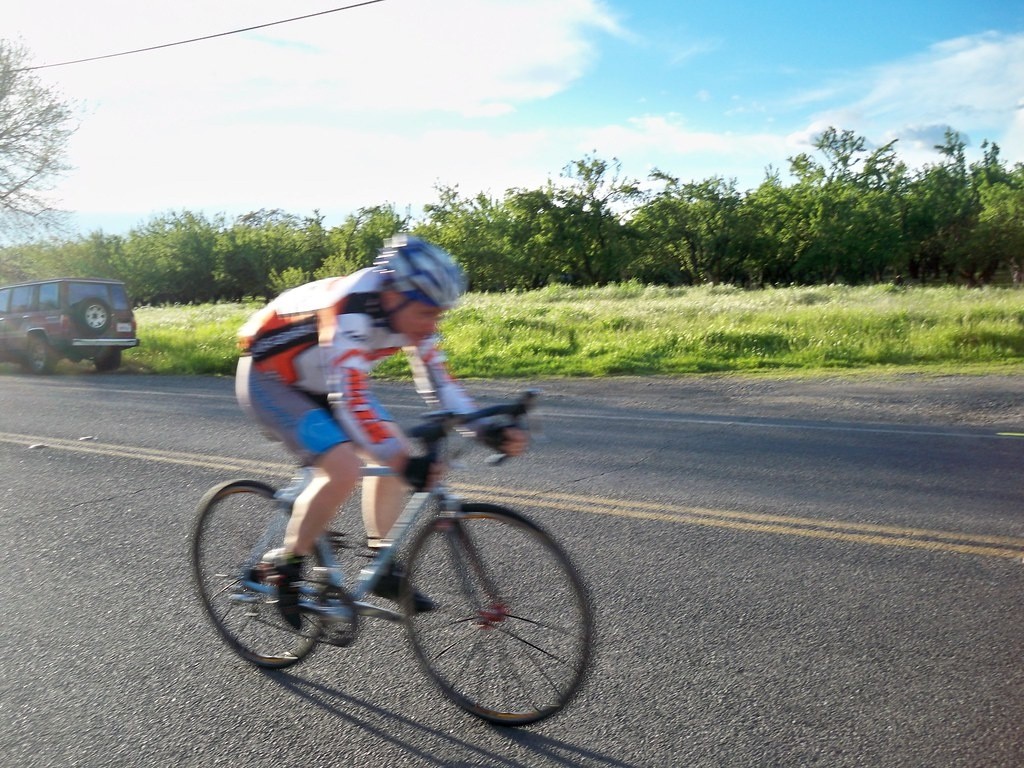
left=235, top=236, right=528, bottom=630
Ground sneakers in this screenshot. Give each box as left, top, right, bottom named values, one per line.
left=358, top=564, right=433, bottom=613
left=273, top=555, right=306, bottom=629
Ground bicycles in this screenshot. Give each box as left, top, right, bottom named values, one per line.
left=186, top=386, right=594, bottom=727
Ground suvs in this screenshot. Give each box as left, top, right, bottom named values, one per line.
left=0, top=277, right=141, bottom=375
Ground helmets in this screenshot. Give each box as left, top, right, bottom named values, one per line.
left=373, top=235, right=469, bottom=310
